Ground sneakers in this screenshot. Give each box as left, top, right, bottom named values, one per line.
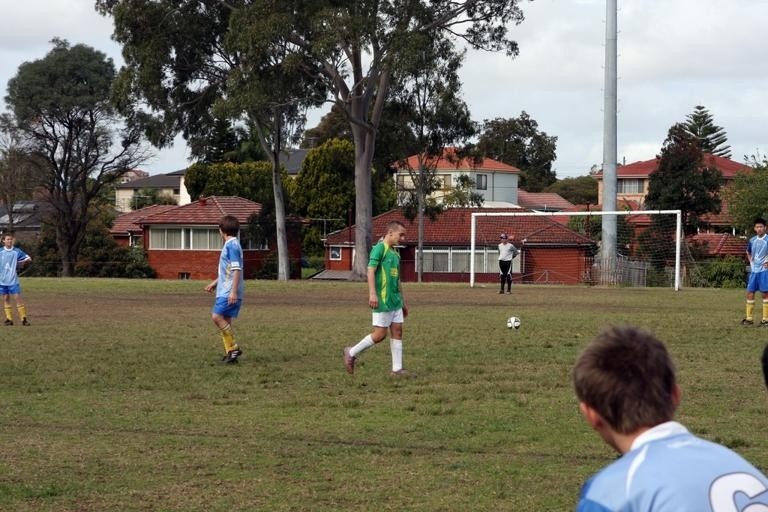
left=757, top=320, right=768, bottom=328
left=4, top=319, right=14, bottom=326
left=739, top=319, right=753, bottom=327
left=23, top=317, right=30, bottom=326
left=343, top=346, right=356, bottom=373
left=221, top=348, right=242, bottom=364
left=390, top=368, right=416, bottom=379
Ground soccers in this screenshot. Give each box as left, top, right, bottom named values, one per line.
left=508, top=316, right=521, bottom=330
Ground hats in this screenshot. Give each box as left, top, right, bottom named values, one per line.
left=500, top=233, right=507, bottom=238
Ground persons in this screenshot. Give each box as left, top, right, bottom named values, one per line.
left=569, top=325, right=767, bottom=512
left=0, top=233, right=33, bottom=326
left=496, top=233, right=518, bottom=295
left=342, top=220, right=415, bottom=380
left=741, top=217, right=767, bottom=325
left=202, top=217, right=244, bottom=364
